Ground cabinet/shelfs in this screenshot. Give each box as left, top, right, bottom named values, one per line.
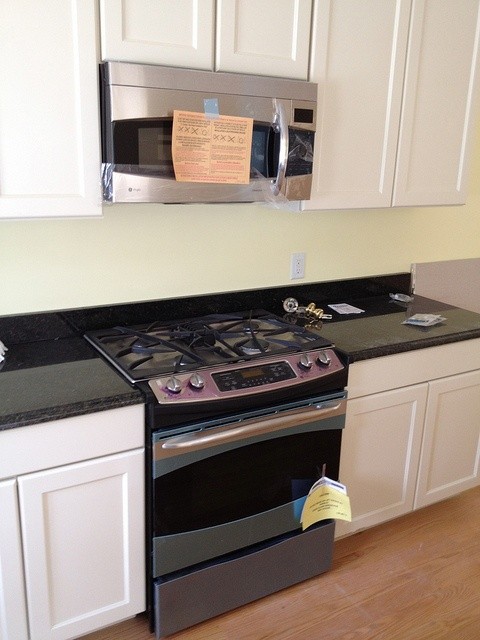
left=0, top=0, right=99, bottom=222
left=99, top=2, right=315, bottom=83
left=331, top=337, right=480, bottom=545
left=300, top=0, right=478, bottom=213
left=1, top=402, right=147, bottom=640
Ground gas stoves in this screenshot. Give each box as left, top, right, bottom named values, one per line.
left=83, top=308, right=351, bottom=434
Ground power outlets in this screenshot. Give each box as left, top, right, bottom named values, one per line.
left=290, top=252, right=306, bottom=279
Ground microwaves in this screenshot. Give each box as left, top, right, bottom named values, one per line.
left=100, top=60, right=319, bottom=205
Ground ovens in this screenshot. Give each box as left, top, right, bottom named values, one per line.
left=145, top=393, right=351, bottom=639
